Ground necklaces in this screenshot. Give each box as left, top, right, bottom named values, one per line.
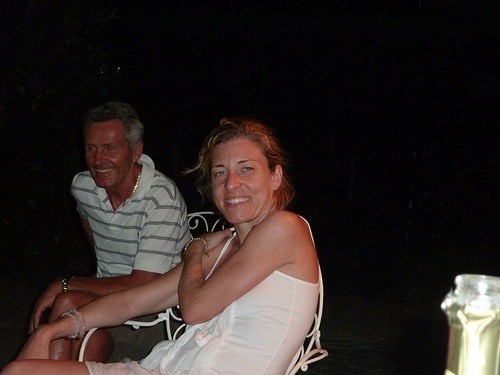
left=131, top=174, right=141, bottom=196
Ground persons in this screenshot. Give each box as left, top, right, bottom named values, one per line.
left=0, top=117, right=319, bottom=375
left=28, top=101, right=192, bottom=363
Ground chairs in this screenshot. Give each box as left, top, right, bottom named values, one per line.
left=79, top=211, right=330, bottom=375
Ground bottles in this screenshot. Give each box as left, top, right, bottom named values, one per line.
left=444, top=275, right=500, bottom=375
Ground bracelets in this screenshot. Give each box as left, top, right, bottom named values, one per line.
left=184, top=238, right=208, bottom=260
left=60, top=309, right=86, bottom=340
left=61, top=275, right=73, bottom=292
